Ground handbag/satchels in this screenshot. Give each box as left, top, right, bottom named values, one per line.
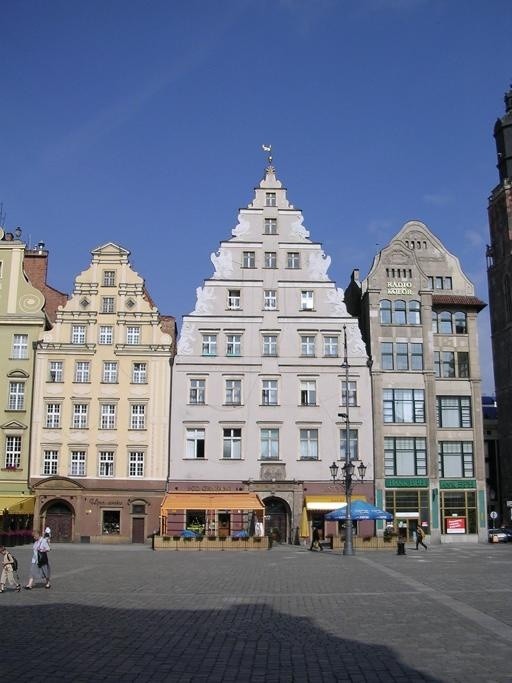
left=37, top=550, right=48, bottom=565
left=312, top=541, right=319, bottom=548
left=12, top=557, right=19, bottom=571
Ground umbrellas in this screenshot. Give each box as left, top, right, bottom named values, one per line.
left=324, top=500, right=393, bottom=520
left=299, top=507, right=310, bottom=548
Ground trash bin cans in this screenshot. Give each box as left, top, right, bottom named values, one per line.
left=397, top=542, right=406, bottom=555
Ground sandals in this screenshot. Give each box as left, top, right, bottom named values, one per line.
left=0, top=582, right=52, bottom=593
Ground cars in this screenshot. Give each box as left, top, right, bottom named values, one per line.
left=488, top=529, right=512, bottom=542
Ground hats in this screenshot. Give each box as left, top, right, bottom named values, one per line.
left=45, top=527, right=51, bottom=533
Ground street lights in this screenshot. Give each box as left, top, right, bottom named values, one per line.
left=330, top=462, right=367, bottom=555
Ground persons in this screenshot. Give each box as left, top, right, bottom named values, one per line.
left=23, top=530, right=51, bottom=590
left=414, top=525, right=427, bottom=550
left=44, top=527, right=51, bottom=551
left=0, top=545, right=21, bottom=593
left=307, top=526, right=323, bottom=551
